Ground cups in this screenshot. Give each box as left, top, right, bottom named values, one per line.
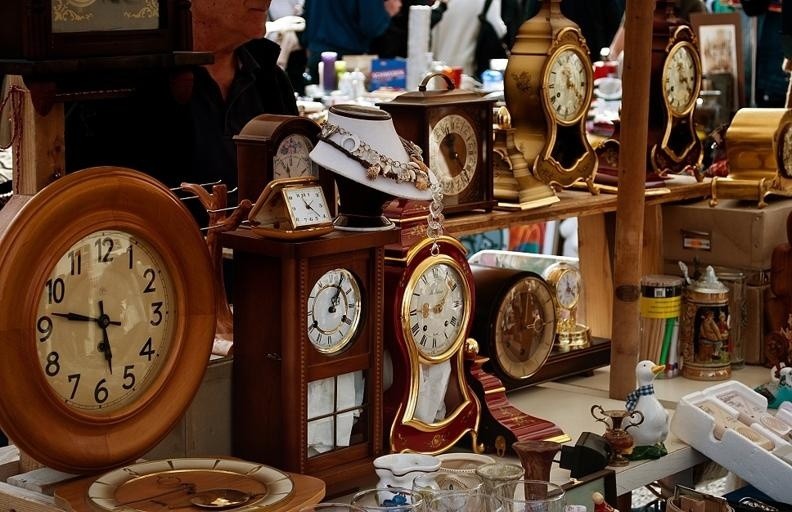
left=301, top=437, right=586, bottom=512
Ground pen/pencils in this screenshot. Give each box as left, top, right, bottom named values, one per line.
left=639, top=275, right=685, bottom=379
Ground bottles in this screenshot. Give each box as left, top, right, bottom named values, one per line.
left=638, top=262, right=733, bottom=381
left=590, top=492, right=619, bottom=512
left=306, top=50, right=463, bottom=108
left=594, top=48, right=618, bottom=79
left=482, top=71, right=504, bottom=91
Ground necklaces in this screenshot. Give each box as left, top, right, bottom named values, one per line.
left=319, top=120, right=445, bottom=264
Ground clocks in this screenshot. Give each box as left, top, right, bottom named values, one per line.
left=0, top=0, right=792, bottom=512
